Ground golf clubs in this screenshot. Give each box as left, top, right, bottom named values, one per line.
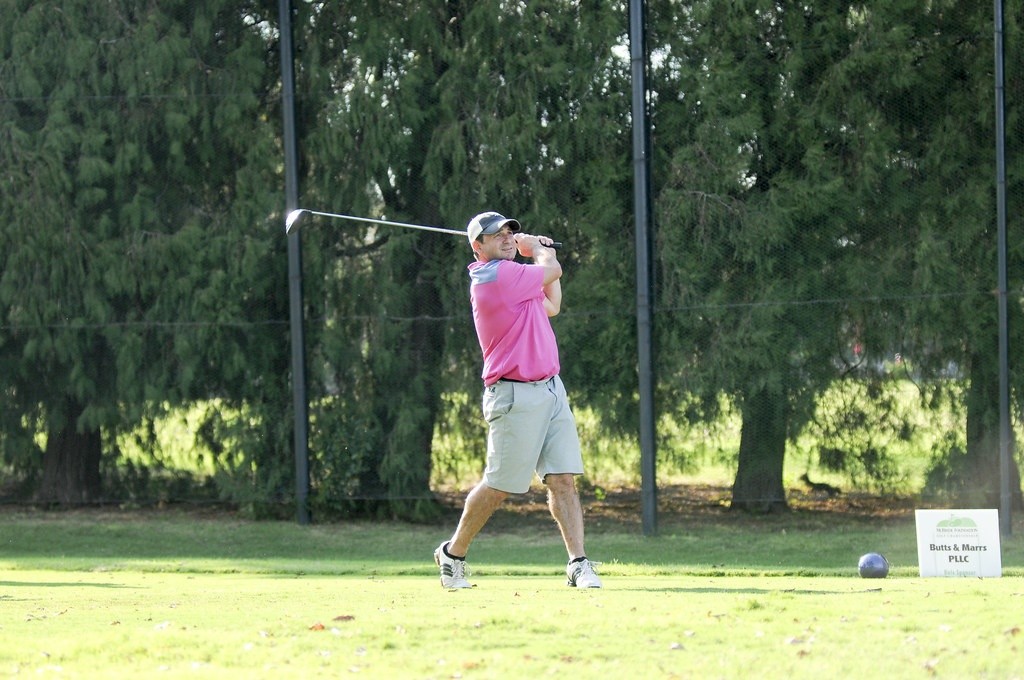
left=284, top=208, right=564, bottom=252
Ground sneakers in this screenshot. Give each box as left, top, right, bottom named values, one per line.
left=434, top=540, right=473, bottom=593
left=564, top=557, right=603, bottom=591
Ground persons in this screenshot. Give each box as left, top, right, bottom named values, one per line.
left=434, top=212, right=603, bottom=590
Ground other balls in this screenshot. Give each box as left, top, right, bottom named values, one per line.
left=858, top=553, right=888, bottom=578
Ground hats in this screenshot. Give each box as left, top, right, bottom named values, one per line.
left=468, top=210, right=521, bottom=246
